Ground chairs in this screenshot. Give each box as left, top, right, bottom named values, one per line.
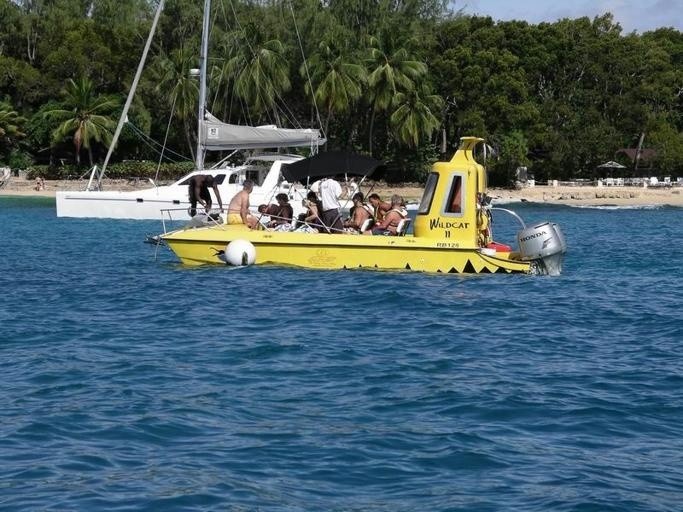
left=395, top=217, right=411, bottom=236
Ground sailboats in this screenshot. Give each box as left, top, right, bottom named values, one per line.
left=54, top=0, right=420, bottom=222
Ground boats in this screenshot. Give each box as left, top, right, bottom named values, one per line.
left=142, top=135, right=567, bottom=276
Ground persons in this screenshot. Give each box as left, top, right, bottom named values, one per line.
left=35, top=175, right=46, bottom=192
left=189, top=174, right=226, bottom=217
left=257, top=170, right=408, bottom=236
left=227, top=179, right=257, bottom=230
